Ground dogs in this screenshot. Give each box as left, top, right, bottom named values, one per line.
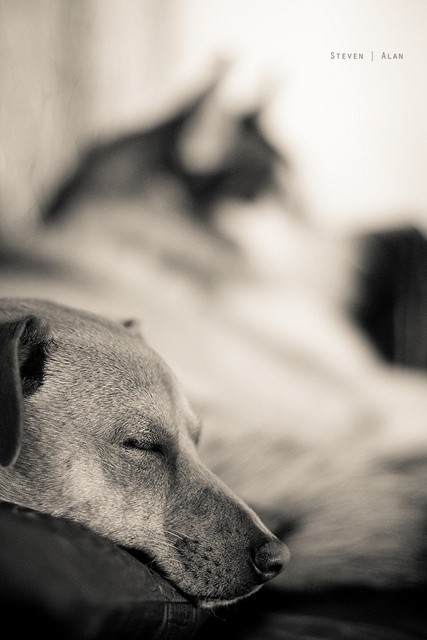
left=0, top=296, right=291, bottom=607
